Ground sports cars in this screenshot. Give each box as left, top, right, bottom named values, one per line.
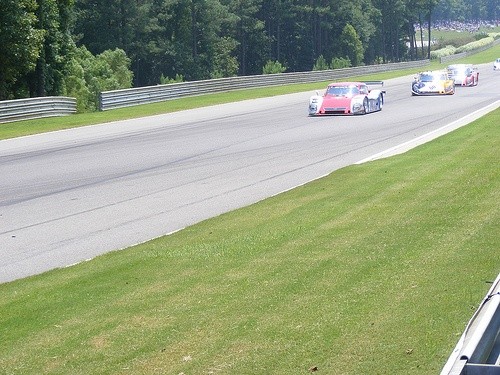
left=494, top=58, right=500, bottom=70
left=443, top=63, right=480, bottom=87
left=411, top=71, right=455, bottom=96
left=308, top=80, right=386, bottom=116
